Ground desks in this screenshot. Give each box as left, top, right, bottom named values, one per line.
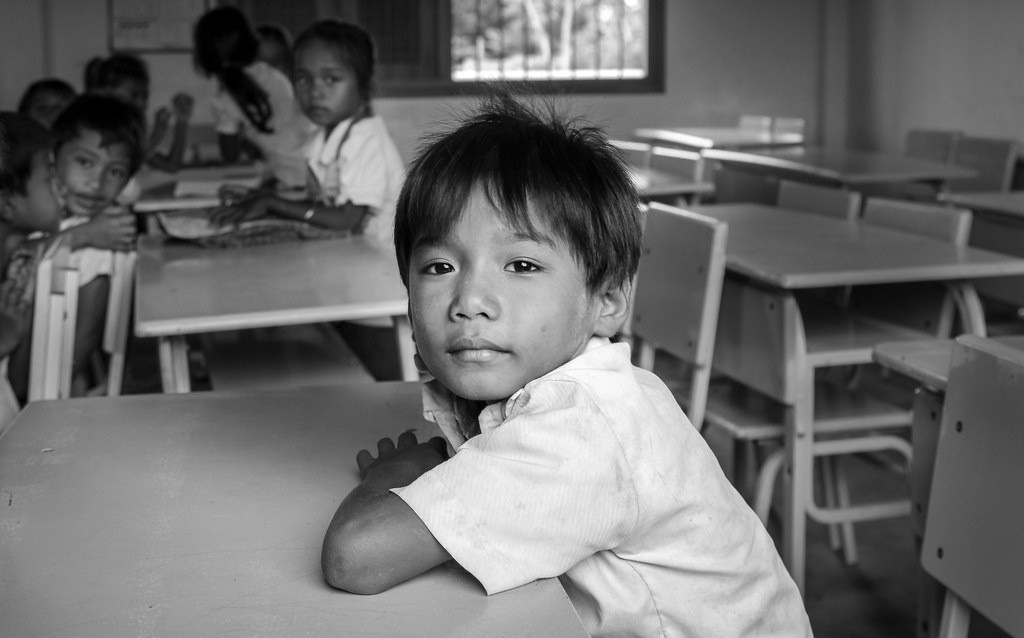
left=700, top=147, right=979, bottom=218
left=935, top=190, right=1024, bottom=308
left=135, top=233, right=419, bottom=394
left=626, top=167, right=714, bottom=205
left=132, top=163, right=266, bottom=233
left=0, top=381, right=591, bottom=638
left=632, top=128, right=803, bottom=153
left=148, top=124, right=222, bottom=166
left=871, top=335, right=1023, bottom=637
left=672, top=202, right=1023, bottom=602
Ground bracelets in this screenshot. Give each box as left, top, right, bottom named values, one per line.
left=305, top=205, right=316, bottom=221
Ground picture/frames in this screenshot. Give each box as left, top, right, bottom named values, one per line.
left=105, top=0, right=210, bottom=53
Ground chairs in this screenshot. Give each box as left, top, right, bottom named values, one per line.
left=0, top=115, right=1024, bottom=637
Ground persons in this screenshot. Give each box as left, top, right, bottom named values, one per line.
left=0, top=7, right=408, bottom=407
left=321, top=97, right=813, bottom=638
